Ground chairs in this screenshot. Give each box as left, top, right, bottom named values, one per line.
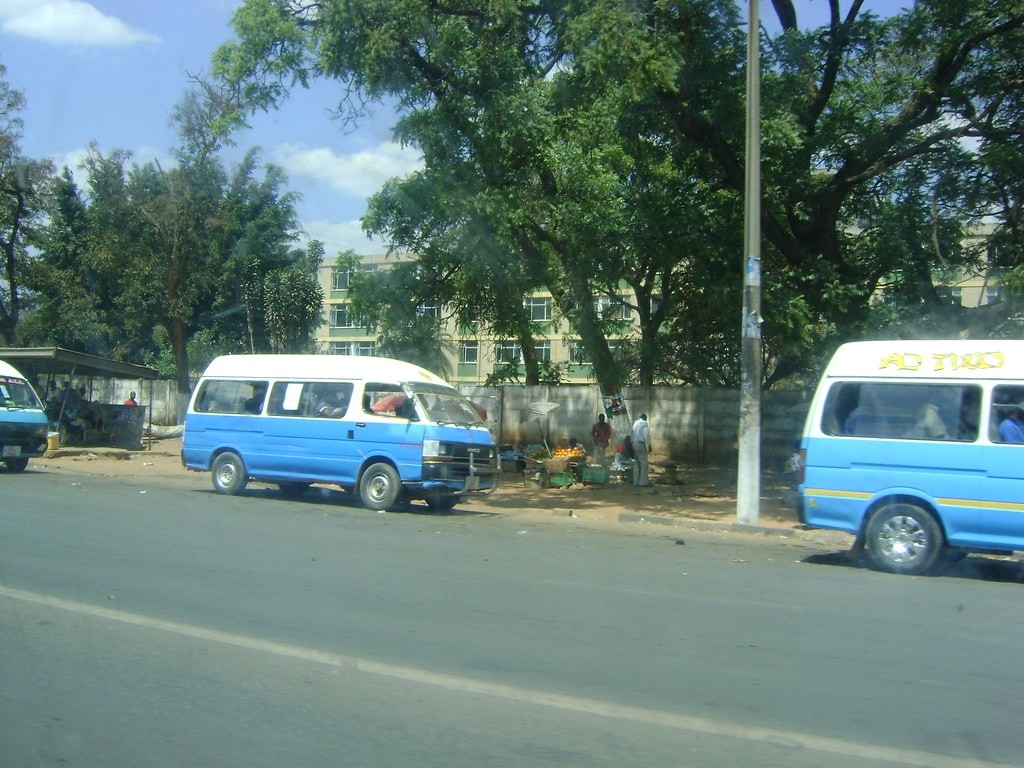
left=309, top=394, right=318, bottom=418
left=988, top=411, right=1004, bottom=441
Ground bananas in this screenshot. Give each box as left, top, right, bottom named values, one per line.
left=529, top=446, right=551, bottom=461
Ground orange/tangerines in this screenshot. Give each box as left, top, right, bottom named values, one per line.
left=553, top=446, right=584, bottom=457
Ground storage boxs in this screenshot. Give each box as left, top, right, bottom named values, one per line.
left=545, top=459, right=574, bottom=486
left=581, top=466, right=610, bottom=483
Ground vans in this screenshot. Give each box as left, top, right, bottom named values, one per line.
left=797, top=338, right=1024, bottom=577
left=181, top=354, right=503, bottom=512
left=0, top=361, right=49, bottom=474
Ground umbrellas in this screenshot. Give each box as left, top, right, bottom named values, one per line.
left=449, top=401, right=487, bottom=421
left=520, top=402, right=559, bottom=457
left=373, top=394, right=405, bottom=412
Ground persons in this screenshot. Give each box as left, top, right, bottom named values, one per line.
left=124, top=392, right=139, bottom=405
left=845, top=392, right=1024, bottom=443
left=632, top=415, right=652, bottom=486
left=314, top=390, right=344, bottom=418
left=568, top=438, right=586, bottom=478
left=593, top=413, right=611, bottom=465
left=243, top=385, right=267, bottom=415
left=616, top=435, right=633, bottom=481
left=34, top=380, right=105, bottom=443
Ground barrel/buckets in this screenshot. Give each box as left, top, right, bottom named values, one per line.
left=48, top=432, right=59, bottom=449
